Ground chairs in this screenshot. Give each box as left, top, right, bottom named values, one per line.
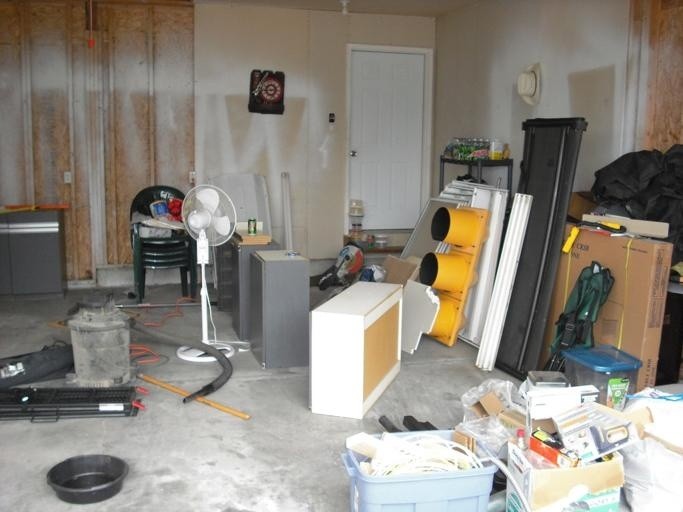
left=128, top=182, right=197, bottom=302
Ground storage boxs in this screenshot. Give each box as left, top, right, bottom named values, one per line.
left=536, top=219, right=674, bottom=410
left=338, top=387, right=683, bottom=512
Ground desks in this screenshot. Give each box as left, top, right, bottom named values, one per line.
left=216, top=232, right=280, bottom=342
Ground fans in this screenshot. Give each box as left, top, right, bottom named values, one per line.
left=176, top=184, right=252, bottom=364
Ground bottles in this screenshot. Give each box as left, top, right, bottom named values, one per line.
left=516, top=429, right=528, bottom=451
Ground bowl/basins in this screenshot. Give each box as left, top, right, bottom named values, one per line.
left=47, top=454, right=129, bottom=504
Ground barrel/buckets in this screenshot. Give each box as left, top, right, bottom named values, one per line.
left=68, top=310, right=139, bottom=386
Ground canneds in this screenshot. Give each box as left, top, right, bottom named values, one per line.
left=248, top=218, right=257, bottom=236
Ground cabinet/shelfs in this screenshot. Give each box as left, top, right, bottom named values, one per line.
left=308, top=280, right=404, bottom=420
left=249, top=249, right=313, bottom=370
left=0, top=209, right=70, bottom=303
left=437, top=155, right=513, bottom=206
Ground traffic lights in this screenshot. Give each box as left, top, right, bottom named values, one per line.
left=417, top=205, right=490, bottom=349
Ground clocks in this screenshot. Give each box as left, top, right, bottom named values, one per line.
left=247, top=68, right=286, bottom=116
